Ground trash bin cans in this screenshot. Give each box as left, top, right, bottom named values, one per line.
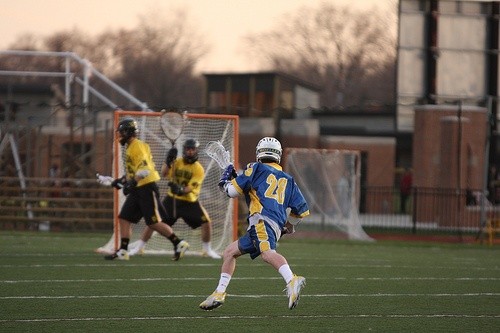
left=63, top=143, right=91, bottom=170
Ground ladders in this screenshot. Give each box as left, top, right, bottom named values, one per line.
left=0, top=133, right=34, bottom=230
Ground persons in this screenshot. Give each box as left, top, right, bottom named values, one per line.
left=198, top=137, right=310, bottom=311
left=128, top=138, right=222, bottom=260
left=104, top=120, right=188, bottom=262
left=400, top=169, right=412, bottom=213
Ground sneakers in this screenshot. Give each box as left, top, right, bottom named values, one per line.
left=129, top=246, right=144, bottom=256
left=104, top=249, right=129, bottom=260
left=282, top=273, right=307, bottom=310
left=199, top=289, right=227, bottom=312
left=201, top=248, right=222, bottom=259
left=170, top=240, right=190, bottom=260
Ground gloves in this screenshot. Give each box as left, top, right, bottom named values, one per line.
left=111, top=175, right=128, bottom=190
left=218, top=164, right=238, bottom=187
left=123, top=176, right=138, bottom=196
left=166, top=148, right=177, bottom=168
left=167, top=181, right=183, bottom=197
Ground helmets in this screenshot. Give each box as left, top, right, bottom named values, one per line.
left=255, top=137, right=282, bottom=164
left=183, top=139, right=200, bottom=164
left=116, top=120, right=139, bottom=145
